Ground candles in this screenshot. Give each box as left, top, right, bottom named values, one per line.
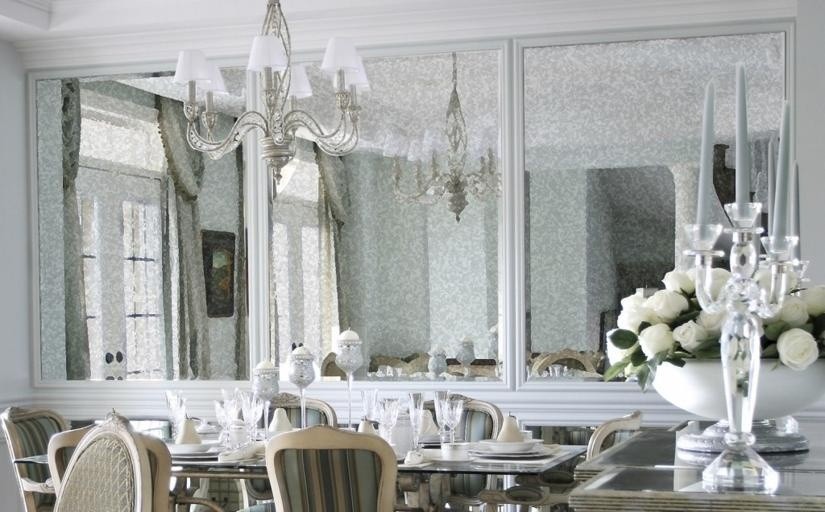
left=693, top=61, right=791, bottom=240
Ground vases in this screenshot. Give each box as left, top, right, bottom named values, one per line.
left=652, top=358, right=819, bottom=453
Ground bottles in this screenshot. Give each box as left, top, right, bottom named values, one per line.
left=496, top=416, right=525, bottom=446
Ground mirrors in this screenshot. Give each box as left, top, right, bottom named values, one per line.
left=20, top=31, right=513, bottom=394
left=514, top=14, right=798, bottom=392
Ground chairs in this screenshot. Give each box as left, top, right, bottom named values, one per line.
left=235, top=393, right=339, bottom=511
left=261, top=423, right=400, bottom=512
left=0, top=402, right=66, bottom=510
left=315, top=349, right=603, bottom=378
left=422, top=389, right=504, bottom=512
left=475, top=409, right=645, bottom=510
left=43, top=410, right=172, bottom=510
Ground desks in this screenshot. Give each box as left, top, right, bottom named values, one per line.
left=572, top=416, right=824, bottom=510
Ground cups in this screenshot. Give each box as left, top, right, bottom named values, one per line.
left=427, top=349, right=447, bottom=377
left=442, top=444, right=469, bottom=461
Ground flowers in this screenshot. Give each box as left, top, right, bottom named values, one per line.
left=604, top=259, right=825, bottom=377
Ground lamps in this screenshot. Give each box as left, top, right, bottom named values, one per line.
left=388, top=53, right=503, bottom=220
left=174, top=0, right=362, bottom=184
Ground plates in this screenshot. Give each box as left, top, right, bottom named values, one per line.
left=475, top=453, right=549, bottom=461
left=164, top=439, right=215, bottom=454
left=471, top=437, right=548, bottom=450
left=431, top=457, right=475, bottom=465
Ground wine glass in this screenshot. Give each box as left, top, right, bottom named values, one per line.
left=238, top=390, right=262, bottom=446
left=163, top=391, right=186, bottom=442
left=218, top=388, right=237, bottom=444
left=291, top=342, right=321, bottom=427
left=337, top=326, right=365, bottom=430
left=356, top=386, right=466, bottom=466
left=249, top=363, right=281, bottom=439
left=456, top=344, right=475, bottom=377
